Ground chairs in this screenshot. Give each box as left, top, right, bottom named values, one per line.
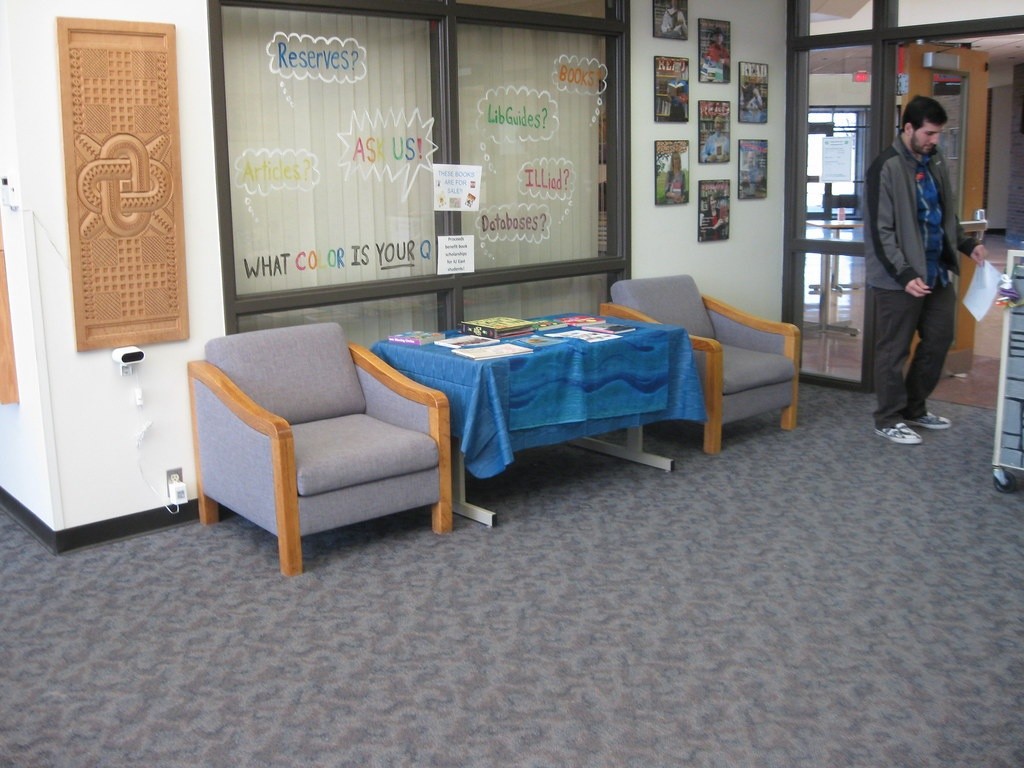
left=599, top=274, right=800, bottom=454
left=187, top=322, right=454, bottom=576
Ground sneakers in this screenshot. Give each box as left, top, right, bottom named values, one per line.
left=874, top=419, right=923, bottom=444
left=905, top=412, right=950, bottom=428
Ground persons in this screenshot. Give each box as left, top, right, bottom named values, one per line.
left=667, top=63, right=688, bottom=123
left=700, top=101, right=729, bottom=119
left=702, top=194, right=728, bottom=241
left=702, top=116, right=730, bottom=163
left=860, top=94, right=989, bottom=444
left=661, top=0, right=688, bottom=38
left=664, top=152, right=686, bottom=202
left=709, top=26, right=730, bottom=62
left=742, top=82, right=766, bottom=112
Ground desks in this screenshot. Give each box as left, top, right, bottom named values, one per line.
left=804, top=219, right=861, bottom=337
left=369, top=314, right=709, bottom=528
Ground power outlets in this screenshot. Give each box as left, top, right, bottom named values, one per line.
left=167, top=467, right=183, bottom=498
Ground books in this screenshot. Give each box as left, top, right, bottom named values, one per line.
left=669, top=182, right=681, bottom=195
left=461, top=316, right=539, bottom=338
left=452, top=343, right=534, bottom=360
left=388, top=330, right=445, bottom=346
left=433, top=334, right=501, bottom=349
left=553, top=315, right=606, bottom=327
left=667, top=83, right=684, bottom=96
left=530, top=318, right=568, bottom=331
left=581, top=322, right=636, bottom=334
